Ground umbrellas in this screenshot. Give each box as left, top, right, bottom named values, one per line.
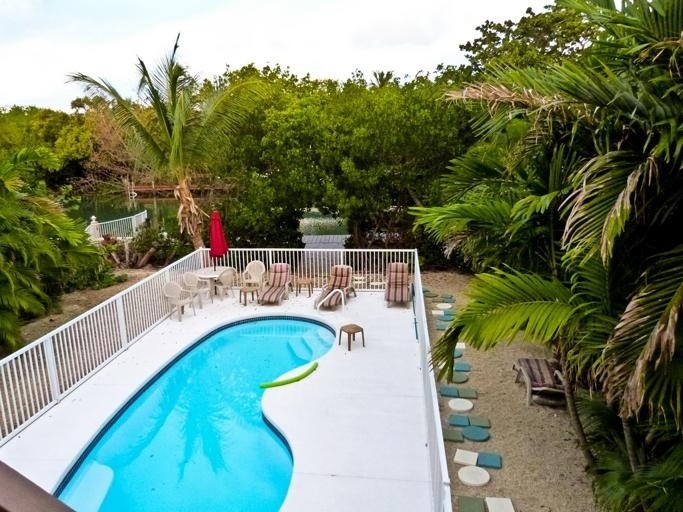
left=209, top=208, right=228, bottom=296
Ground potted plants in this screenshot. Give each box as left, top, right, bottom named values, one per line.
left=298, top=217, right=352, bottom=273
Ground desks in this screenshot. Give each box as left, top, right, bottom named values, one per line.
left=196, top=266, right=236, bottom=295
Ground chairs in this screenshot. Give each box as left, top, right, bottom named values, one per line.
left=314, top=265, right=356, bottom=310
left=384, top=263, right=413, bottom=309
left=162, top=281, right=196, bottom=322
left=258, top=263, right=293, bottom=306
left=240, top=260, right=265, bottom=287
left=512, top=358, right=572, bottom=407
left=214, top=268, right=237, bottom=301
left=183, top=272, right=213, bottom=309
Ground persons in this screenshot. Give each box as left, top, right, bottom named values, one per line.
left=364, top=228, right=404, bottom=248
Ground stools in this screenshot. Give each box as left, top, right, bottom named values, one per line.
left=296, top=279, right=313, bottom=297
left=239, top=287, right=260, bottom=306
left=338, top=325, right=365, bottom=351
